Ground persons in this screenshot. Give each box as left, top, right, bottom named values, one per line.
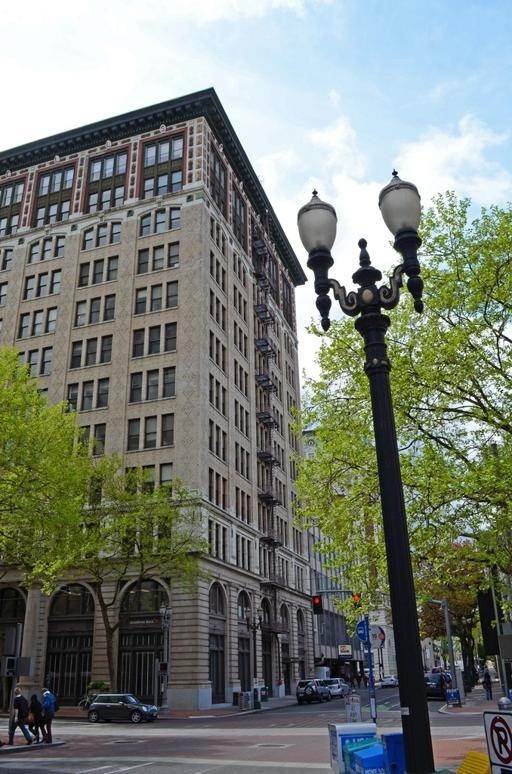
left=484, top=665, right=489, bottom=672
left=481, top=669, right=493, bottom=700
left=340, top=671, right=349, bottom=682
left=28, top=693, right=41, bottom=743
left=8, top=687, right=33, bottom=745
left=363, top=673, right=368, bottom=688
left=357, top=673, right=361, bottom=689
left=350, top=672, right=356, bottom=689
left=40, top=687, right=56, bottom=743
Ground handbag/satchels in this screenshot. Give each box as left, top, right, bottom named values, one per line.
left=24, top=712, right=34, bottom=724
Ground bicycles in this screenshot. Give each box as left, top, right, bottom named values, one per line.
left=78, top=692, right=97, bottom=713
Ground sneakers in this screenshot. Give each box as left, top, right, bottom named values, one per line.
left=6, top=741, right=13, bottom=745
left=27, top=734, right=51, bottom=744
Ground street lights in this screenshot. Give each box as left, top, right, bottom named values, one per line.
left=159, top=600, right=172, bottom=715
left=352, top=592, right=362, bottom=610
left=245, top=604, right=263, bottom=710
left=297, top=168, right=454, bottom=774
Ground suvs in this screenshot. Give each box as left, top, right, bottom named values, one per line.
left=296, top=678, right=332, bottom=705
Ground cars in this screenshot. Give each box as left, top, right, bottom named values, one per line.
left=88, top=693, right=158, bottom=724
left=323, top=678, right=350, bottom=698
left=381, top=674, right=400, bottom=689
left=424, top=665, right=466, bottom=700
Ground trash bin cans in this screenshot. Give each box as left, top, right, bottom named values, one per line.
left=339, top=733, right=409, bottom=774
left=261, top=687, right=269, bottom=702
left=233, top=692, right=239, bottom=706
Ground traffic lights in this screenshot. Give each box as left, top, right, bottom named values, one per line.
left=312, top=594, right=323, bottom=615
